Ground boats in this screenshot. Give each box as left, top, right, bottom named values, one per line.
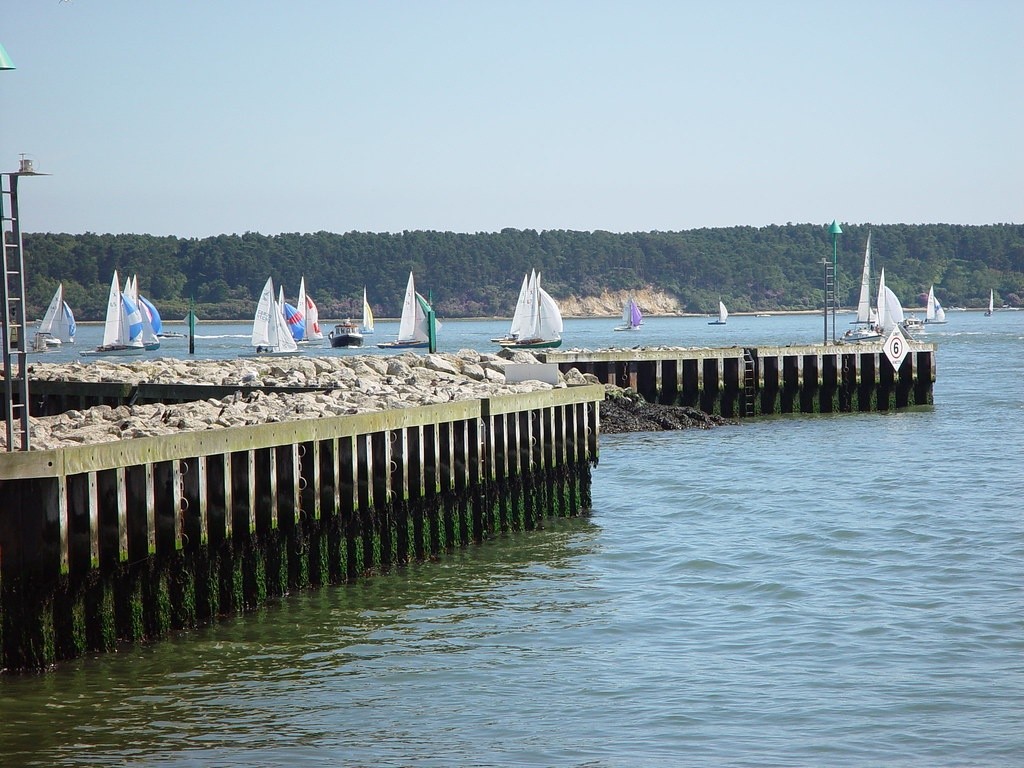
left=328, top=317, right=363, bottom=348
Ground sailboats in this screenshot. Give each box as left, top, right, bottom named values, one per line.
left=78, top=270, right=187, bottom=358
left=29, top=283, right=76, bottom=347
left=708, top=295, right=729, bottom=325
left=376, top=270, right=443, bottom=349
left=491, top=268, right=563, bottom=349
left=613, top=298, right=644, bottom=331
left=359, top=284, right=375, bottom=334
left=237, top=268, right=323, bottom=357
left=921, top=285, right=949, bottom=324
left=840, top=225, right=926, bottom=343
left=984, top=288, right=994, bottom=316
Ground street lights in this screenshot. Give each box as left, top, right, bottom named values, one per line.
left=824, top=218, right=843, bottom=347
